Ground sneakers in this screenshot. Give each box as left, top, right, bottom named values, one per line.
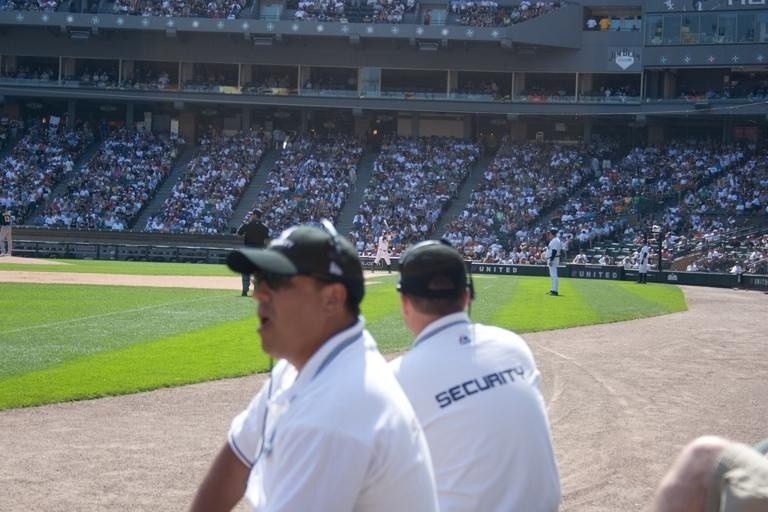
left=242, top=288, right=249, bottom=296
left=548, top=291, right=558, bottom=296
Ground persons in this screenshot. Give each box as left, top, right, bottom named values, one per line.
left=1, top=1, right=766, bottom=44
left=634, top=238, right=649, bottom=284
left=188, top=221, right=443, bottom=512
left=639, top=431, right=768, bottom=512
left=1, top=97, right=767, bottom=271
left=237, top=208, right=270, bottom=297
left=27, top=56, right=767, bottom=103
left=387, top=239, right=564, bottom=512
left=544, top=227, right=561, bottom=296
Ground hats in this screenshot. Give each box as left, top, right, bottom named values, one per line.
left=398, top=242, right=466, bottom=297
left=227, top=225, right=363, bottom=280
left=550, top=227, right=558, bottom=234
left=250, top=271, right=292, bottom=290
left=249, top=209, right=262, bottom=216
